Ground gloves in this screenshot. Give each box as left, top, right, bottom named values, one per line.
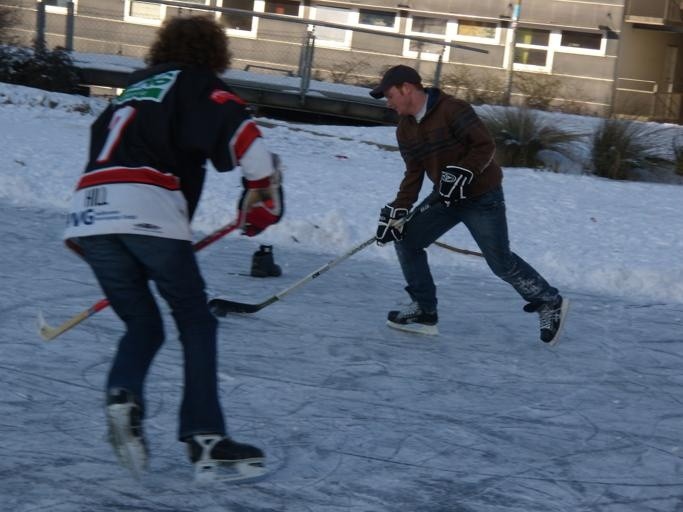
left=238, top=178, right=282, bottom=237
left=438, top=165, right=474, bottom=207
left=376, top=205, right=407, bottom=245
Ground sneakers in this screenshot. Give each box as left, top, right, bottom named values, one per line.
left=524, top=295, right=562, bottom=343
left=188, top=437, right=264, bottom=464
left=106, top=389, right=149, bottom=458
left=388, top=305, right=438, bottom=327
left=252, top=249, right=281, bottom=277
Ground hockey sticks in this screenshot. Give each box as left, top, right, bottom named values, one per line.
left=35, top=200, right=275, bottom=340
left=207, top=195, right=445, bottom=314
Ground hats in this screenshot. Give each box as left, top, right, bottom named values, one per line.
left=370, top=66, right=422, bottom=100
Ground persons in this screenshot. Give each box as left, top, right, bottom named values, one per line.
left=369, top=65, right=569, bottom=346
left=62, top=13, right=284, bottom=469
